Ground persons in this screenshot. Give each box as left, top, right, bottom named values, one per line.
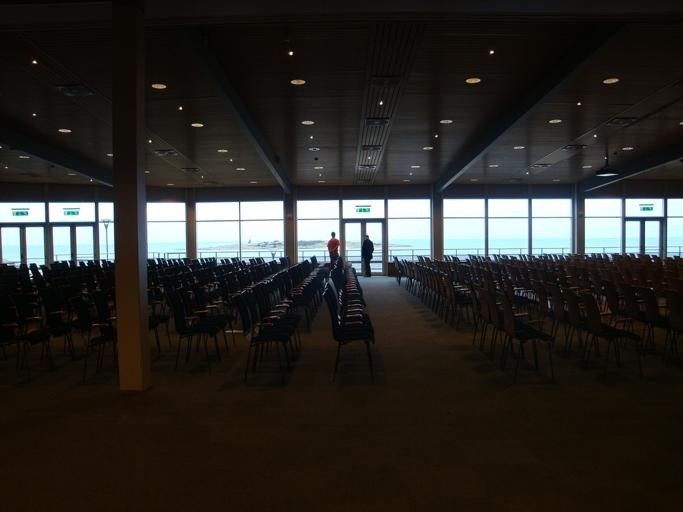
left=361, top=235, right=374, bottom=277
left=326, top=231, right=340, bottom=269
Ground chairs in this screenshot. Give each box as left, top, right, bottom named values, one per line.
left=392, top=251, right=682, bottom=379
left=0, top=258, right=378, bottom=382
